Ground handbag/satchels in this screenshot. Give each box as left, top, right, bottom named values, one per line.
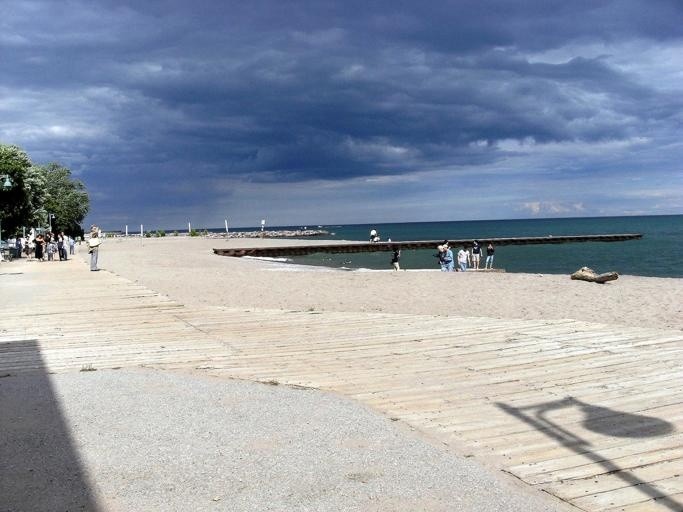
left=437, top=245, right=443, bottom=252
left=89, top=238, right=100, bottom=247
left=444, top=257, right=452, bottom=262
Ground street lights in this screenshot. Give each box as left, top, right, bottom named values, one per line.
left=49, top=213, right=55, bottom=235
left=0, top=174, right=12, bottom=189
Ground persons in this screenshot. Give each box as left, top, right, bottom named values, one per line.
left=432, top=238, right=495, bottom=272
left=391, top=246, right=401, bottom=271
left=0, top=224, right=103, bottom=271
left=372, top=233, right=380, bottom=243
left=368, top=227, right=377, bottom=243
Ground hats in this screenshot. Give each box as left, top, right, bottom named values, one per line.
left=442, top=244, right=448, bottom=247
